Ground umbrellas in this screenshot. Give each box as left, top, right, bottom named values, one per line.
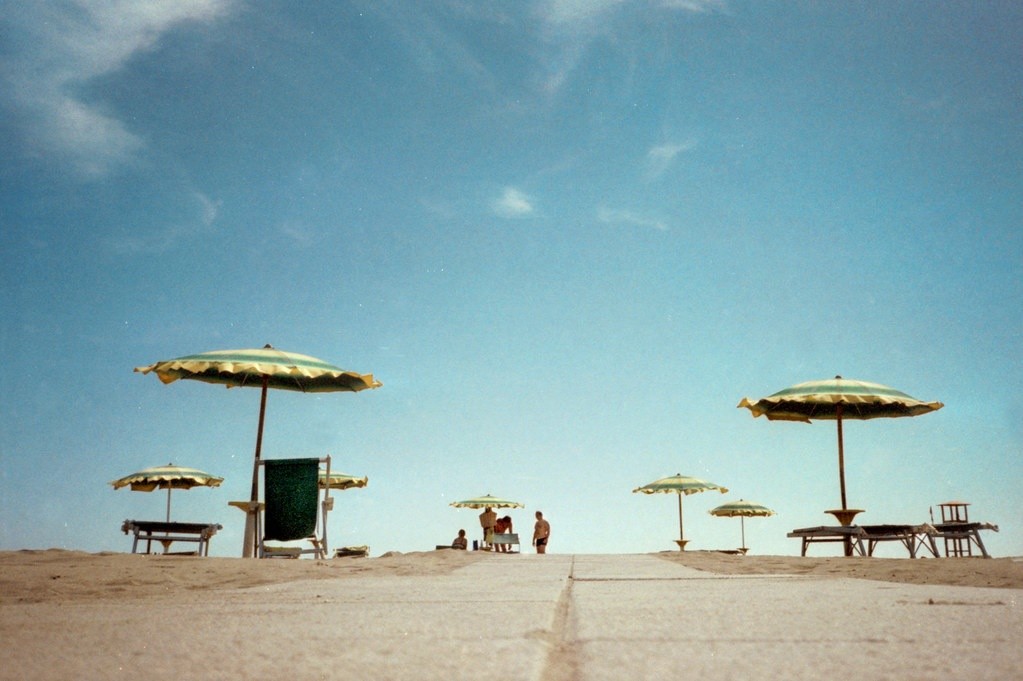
left=110, top=463, right=225, bottom=535
left=709, top=499, right=777, bottom=549
left=134, top=345, right=379, bottom=559
left=737, top=375, right=943, bottom=509
left=449, top=494, right=525, bottom=549
left=633, top=473, right=729, bottom=541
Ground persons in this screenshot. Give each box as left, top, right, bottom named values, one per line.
left=493, top=516, right=512, bottom=552
left=452, top=529, right=466, bottom=550
left=532, top=511, right=550, bottom=554
left=314, top=466, right=369, bottom=538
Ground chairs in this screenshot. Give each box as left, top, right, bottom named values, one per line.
left=787, top=522, right=998, bottom=557
left=255, top=454, right=331, bottom=560
